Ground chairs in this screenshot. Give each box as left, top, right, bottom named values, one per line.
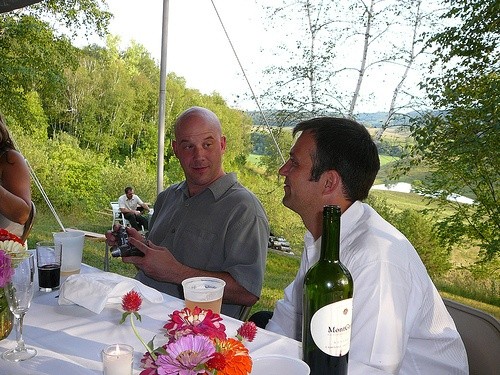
left=443, top=298, right=500, bottom=375
left=110, top=202, right=146, bottom=232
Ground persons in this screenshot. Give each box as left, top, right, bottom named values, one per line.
left=115, top=186, right=153, bottom=236
left=0, top=118, right=31, bottom=248
left=106, top=106, right=270, bottom=320
left=247, top=116, right=470, bottom=375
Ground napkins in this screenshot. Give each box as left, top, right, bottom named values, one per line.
left=57, top=272, right=165, bottom=315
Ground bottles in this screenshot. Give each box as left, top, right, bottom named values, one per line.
left=301, top=203, right=354, bottom=375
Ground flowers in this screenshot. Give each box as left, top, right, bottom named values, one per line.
left=118, top=290, right=258, bottom=375
left=0, top=229, right=36, bottom=288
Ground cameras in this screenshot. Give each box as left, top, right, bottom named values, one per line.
left=109, top=226, right=146, bottom=257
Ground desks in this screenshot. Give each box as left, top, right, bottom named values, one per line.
left=0, top=249, right=394, bottom=375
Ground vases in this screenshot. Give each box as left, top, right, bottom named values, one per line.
left=0, top=283, right=15, bottom=340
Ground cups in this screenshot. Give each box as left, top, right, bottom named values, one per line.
left=36, top=241, right=63, bottom=291
left=249, top=354, right=311, bottom=375
left=181, top=277, right=226, bottom=318
left=54, top=231, right=84, bottom=282
left=102, top=343, right=134, bottom=375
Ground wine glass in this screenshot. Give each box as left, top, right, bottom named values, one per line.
left=2, top=251, right=37, bottom=362
